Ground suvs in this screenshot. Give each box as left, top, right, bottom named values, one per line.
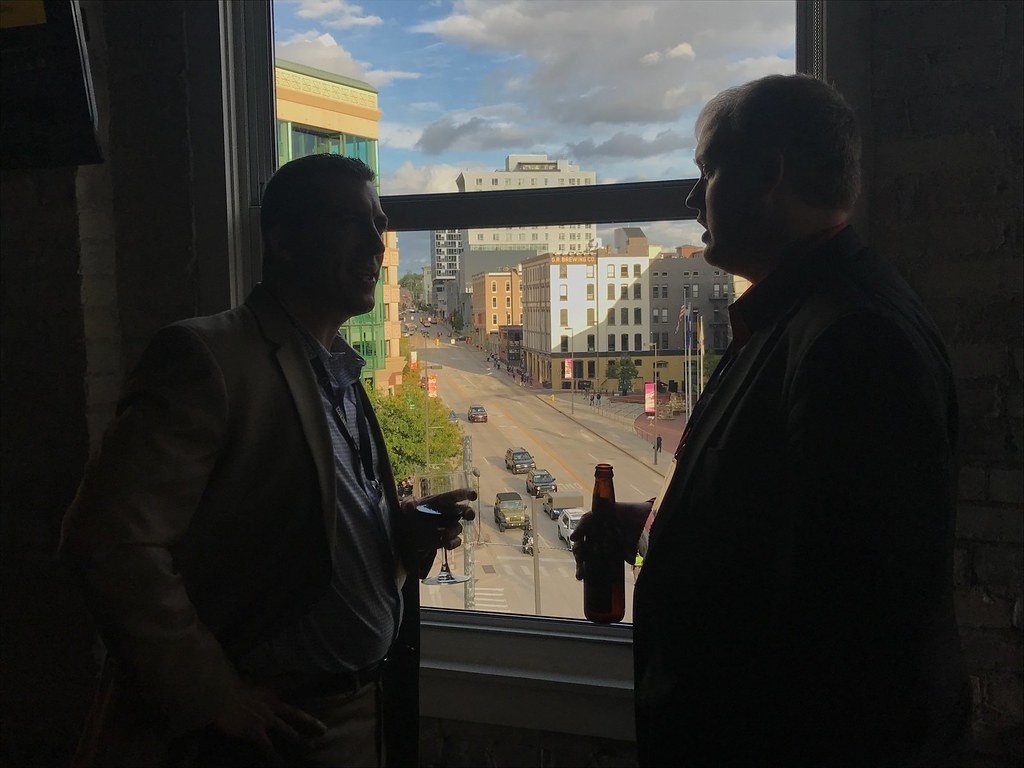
left=544, top=491, right=584, bottom=519
left=494, top=492, right=530, bottom=532
left=468, top=406, right=488, bottom=423
left=557, top=509, right=588, bottom=551
left=503, top=446, right=536, bottom=475
left=527, top=470, right=557, bottom=498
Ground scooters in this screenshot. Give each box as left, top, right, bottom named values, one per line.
left=524, top=533, right=538, bottom=557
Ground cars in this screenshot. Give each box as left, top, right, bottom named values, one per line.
left=455, top=335, right=466, bottom=341
left=421, top=317, right=437, bottom=327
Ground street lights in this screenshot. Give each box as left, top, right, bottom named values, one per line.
left=472, top=465, right=482, bottom=544
left=564, top=326, right=574, bottom=415
left=643, top=341, right=658, bottom=466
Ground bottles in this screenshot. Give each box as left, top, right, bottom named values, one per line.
left=584, top=463, right=625, bottom=624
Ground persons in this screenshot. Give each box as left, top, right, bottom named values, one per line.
left=69, top=154, right=480, bottom=768
left=567, top=71, right=976, bottom=766
left=656, top=433, right=663, bottom=453
left=506, top=366, right=533, bottom=387
left=589, top=393, right=601, bottom=406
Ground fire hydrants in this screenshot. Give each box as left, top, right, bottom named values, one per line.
left=551, top=394, right=554, bottom=402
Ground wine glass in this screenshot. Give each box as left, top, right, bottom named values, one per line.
left=411, top=470, right=473, bottom=584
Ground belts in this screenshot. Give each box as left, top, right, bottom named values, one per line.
left=263, top=658, right=390, bottom=699
